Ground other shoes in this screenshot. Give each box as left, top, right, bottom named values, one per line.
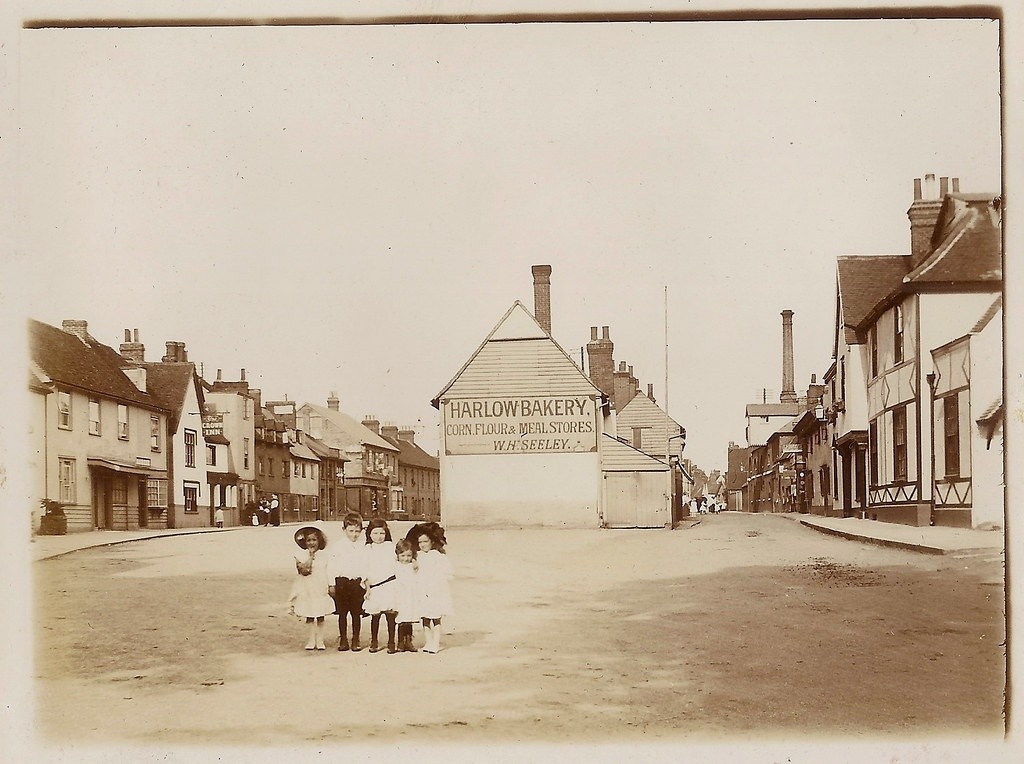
left=369, top=640, right=378, bottom=652
left=338, top=639, right=349, bottom=651
left=305, top=639, right=315, bottom=649
left=316, top=639, right=326, bottom=650
left=387, top=642, right=396, bottom=654
left=352, top=639, right=361, bottom=651
left=397, top=640, right=406, bottom=653
left=406, top=641, right=417, bottom=652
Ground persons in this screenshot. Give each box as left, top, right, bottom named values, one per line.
left=215, top=506, right=223, bottom=528
left=292, top=512, right=454, bottom=654
left=687, top=493, right=727, bottom=518
left=251, top=493, right=280, bottom=527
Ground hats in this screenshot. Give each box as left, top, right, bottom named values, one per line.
left=294, top=526, right=328, bottom=550
left=405, top=522, right=447, bottom=551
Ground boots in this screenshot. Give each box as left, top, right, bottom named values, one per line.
left=428, top=624, right=441, bottom=653
left=423, top=626, right=431, bottom=654
